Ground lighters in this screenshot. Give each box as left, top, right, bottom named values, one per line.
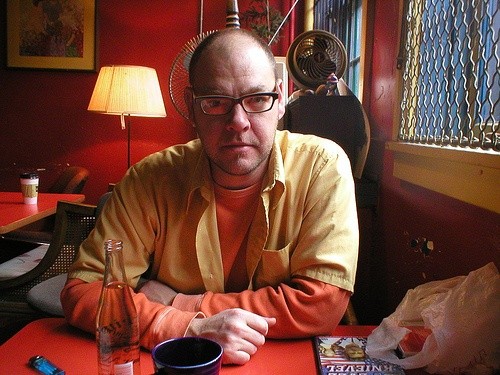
left=30, top=355, right=67, bottom=375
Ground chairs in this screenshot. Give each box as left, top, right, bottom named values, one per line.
left=0, top=166, right=357, bottom=327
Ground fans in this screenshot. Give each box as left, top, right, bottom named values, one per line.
left=285, top=30, right=348, bottom=90
left=168, top=30, right=218, bottom=121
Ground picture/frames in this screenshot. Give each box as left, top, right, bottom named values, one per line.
left=7, top=0, right=95, bottom=70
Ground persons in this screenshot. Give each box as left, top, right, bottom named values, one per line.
left=60, top=29, right=359, bottom=365
left=318, top=337, right=368, bottom=362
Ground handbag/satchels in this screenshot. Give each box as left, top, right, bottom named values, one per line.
left=365, top=262, right=500, bottom=375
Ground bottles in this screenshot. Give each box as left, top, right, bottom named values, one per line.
left=97, top=240, right=141, bottom=375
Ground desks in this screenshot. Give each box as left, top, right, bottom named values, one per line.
left=0, top=317, right=379, bottom=375
left=0, top=191, right=86, bottom=234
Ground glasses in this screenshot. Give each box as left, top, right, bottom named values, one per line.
left=191, top=83, right=283, bottom=116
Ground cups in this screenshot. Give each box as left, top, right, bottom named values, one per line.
left=149, top=337, right=223, bottom=375
left=20, top=172, right=39, bottom=204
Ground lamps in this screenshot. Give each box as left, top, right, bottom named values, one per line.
left=88, top=66, right=167, bottom=169
